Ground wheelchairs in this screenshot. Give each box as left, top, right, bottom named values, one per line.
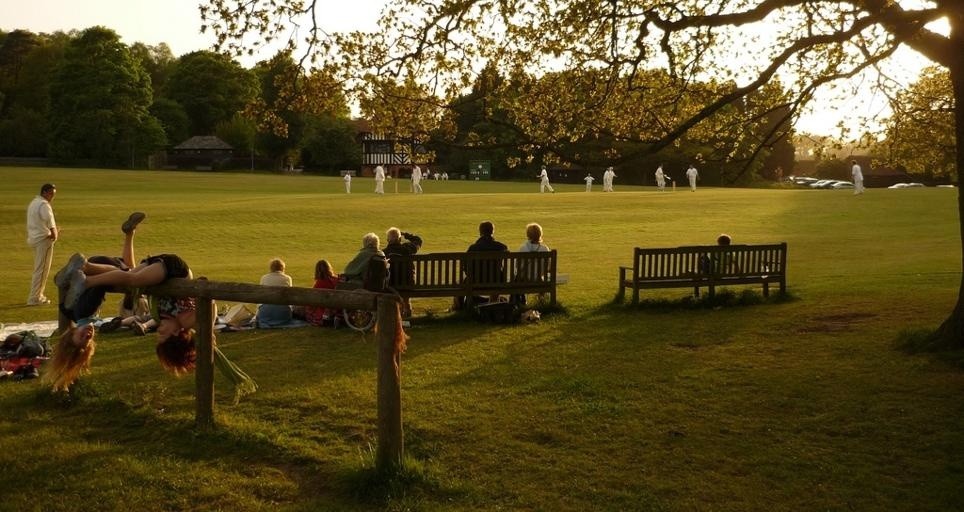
left=332, top=256, right=391, bottom=331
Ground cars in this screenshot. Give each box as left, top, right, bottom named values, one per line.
left=785, top=171, right=866, bottom=189
left=885, top=181, right=955, bottom=190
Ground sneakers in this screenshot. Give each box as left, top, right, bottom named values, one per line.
left=9, top=253, right=147, bottom=380
left=122, top=213, right=146, bottom=233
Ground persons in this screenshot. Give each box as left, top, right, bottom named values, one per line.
left=54, top=253, right=217, bottom=376
left=323, top=232, right=386, bottom=330
left=694, top=234, right=741, bottom=298
left=851, top=160, right=864, bottom=194
left=535, top=163, right=701, bottom=193
left=452, top=222, right=507, bottom=312
left=98, top=314, right=158, bottom=335
left=38, top=212, right=145, bottom=394
left=343, top=161, right=449, bottom=194
left=514, top=223, right=550, bottom=305
left=254, top=259, right=306, bottom=328
left=27, top=183, right=57, bottom=305
left=294, top=260, right=341, bottom=327
left=381, top=227, right=423, bottom=316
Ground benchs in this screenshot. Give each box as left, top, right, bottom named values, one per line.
left=389, top=249, right=558, bottom=315
left=618, top=241, right=787, bottom=304
